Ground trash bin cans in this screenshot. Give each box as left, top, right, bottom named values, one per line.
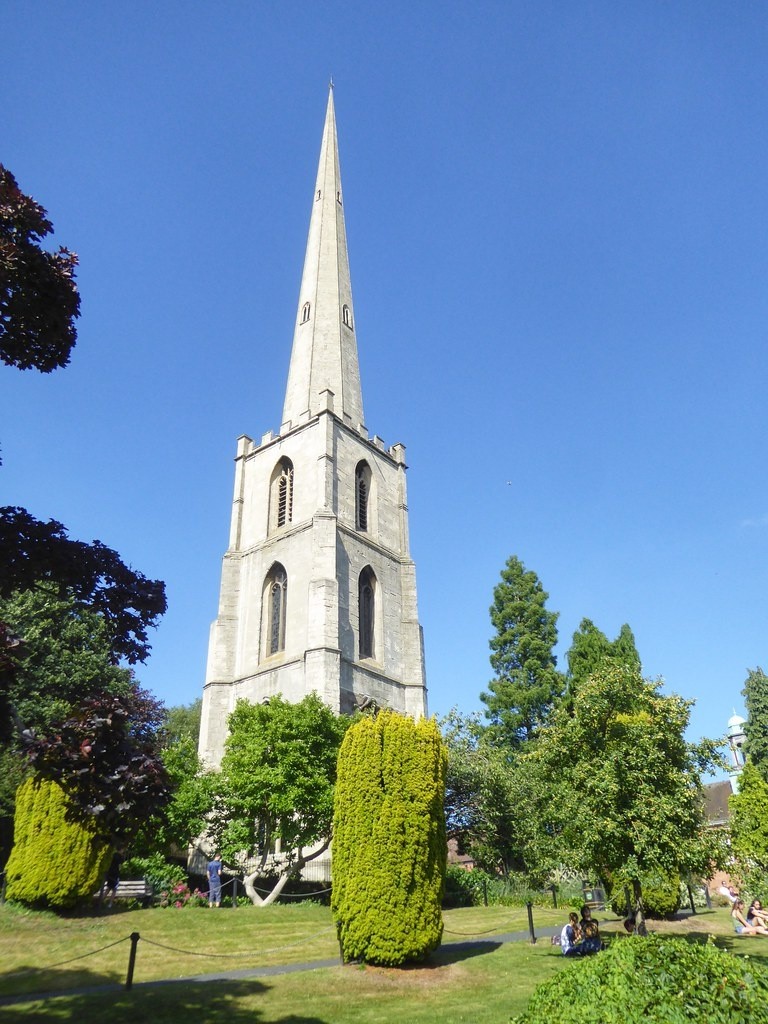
left=582, top=879, right=605, bottom=912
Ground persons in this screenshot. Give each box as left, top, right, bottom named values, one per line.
left=207, top=852, right=223, bottom=908
left=720, top=881, right=768, bottom=936
left=560, top=906, right=607, bottom=955
left=623, top=918, right=637, bottom=937
left=103, top=845, right=125, bottom=908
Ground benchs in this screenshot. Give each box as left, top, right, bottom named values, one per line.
left=90, top=879, right=153, bottom=908
